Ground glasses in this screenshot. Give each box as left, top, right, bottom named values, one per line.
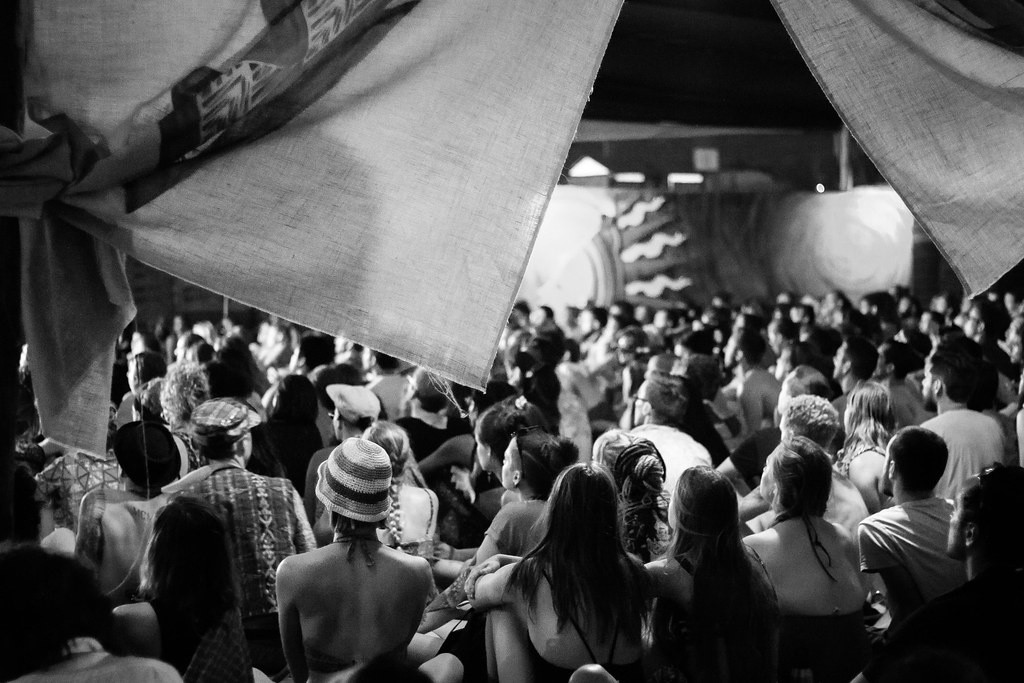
left=980, top=462, right=1005, bottom=507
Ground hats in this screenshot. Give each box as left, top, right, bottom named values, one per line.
left=314, top=437, right=392, bottom=522
left=78, top=504, right=151, bottom=597
left=190, top=395, right=263, bottom=446
left=326, top=385, right=381, bottom=422
left=644, top=354, right=690, bottom=380
left=113, top=421, right=180, bottom=487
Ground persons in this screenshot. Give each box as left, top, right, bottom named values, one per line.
left=0, top=284, right=1024, bottom=683
